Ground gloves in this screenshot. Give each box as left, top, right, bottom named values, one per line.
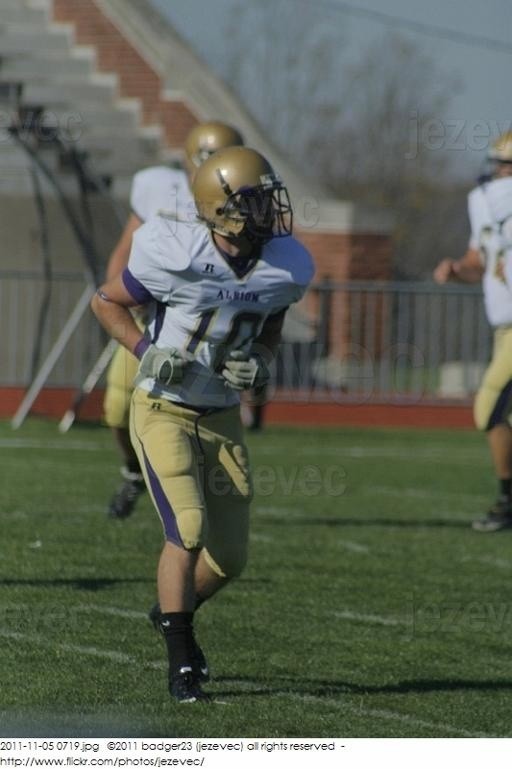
left=222, top=343, right=274, bottom=390
left=139, top=344, right=197, bottom=384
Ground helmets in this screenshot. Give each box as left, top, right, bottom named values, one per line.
left=185, top=123, right=292, bottom=239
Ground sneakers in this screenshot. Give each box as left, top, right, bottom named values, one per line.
left=148, top=601, right=209, bottom=703
left=109, top=465, right=146, bottom=517
left=472, top=499, right=511, bottom=531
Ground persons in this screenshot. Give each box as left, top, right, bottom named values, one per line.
left=88, top=142, right=316, bottom=704
left=99, top=121, right=242, bottom=521
left=433, top=131, right=512, bottom=534
